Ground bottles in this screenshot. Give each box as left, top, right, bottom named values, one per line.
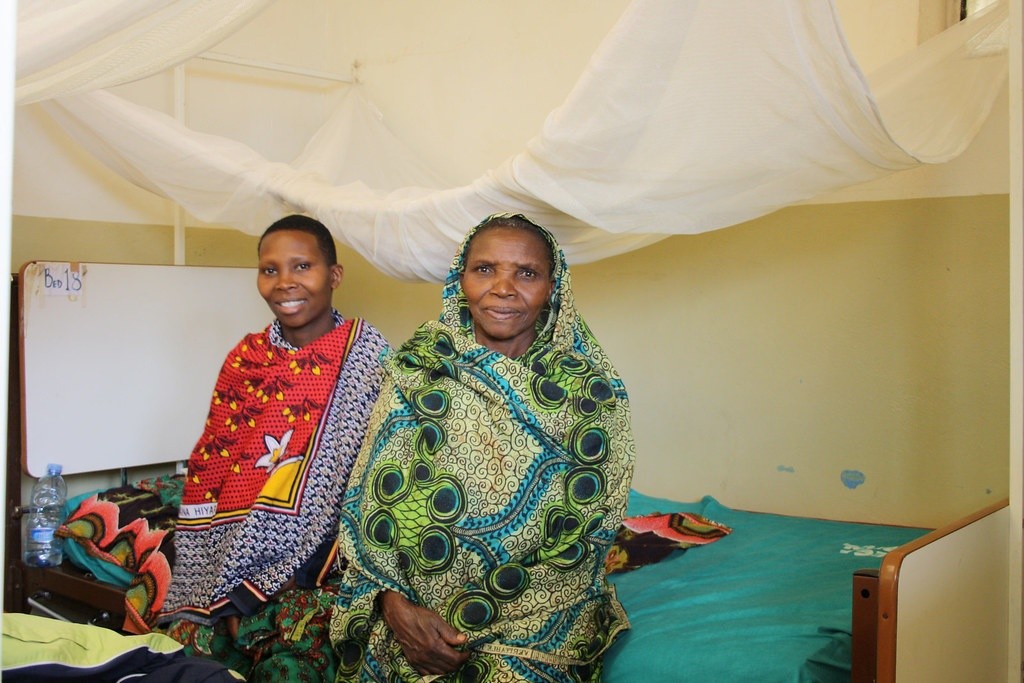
left=24, top=463, right=68, bottom=567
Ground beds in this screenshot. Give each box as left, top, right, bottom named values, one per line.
left=9, top=257, right=1009, bottom=682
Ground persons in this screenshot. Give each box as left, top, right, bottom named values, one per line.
left=328, top=211, right=631, bottom=683
left=156, top=213, right=399, bottom=683
